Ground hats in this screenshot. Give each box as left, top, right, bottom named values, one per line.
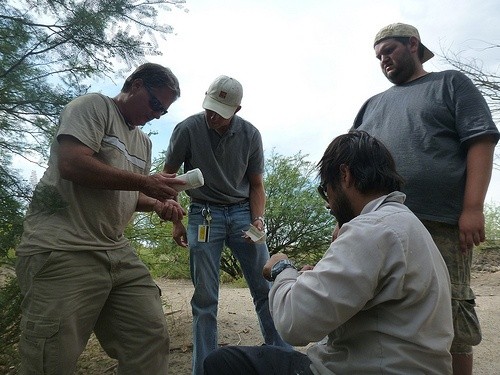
left=374, top=23, right=434, bottom=64
left=202, top=75, right=243, bottom=119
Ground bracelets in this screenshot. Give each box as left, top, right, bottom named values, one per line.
left=252, top=217, right=264, bottom=225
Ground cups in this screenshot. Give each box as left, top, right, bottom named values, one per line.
left=170, top=169, right=204, bottom=192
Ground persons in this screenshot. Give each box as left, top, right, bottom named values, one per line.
left=203, top=132, right=455, bottom=375
left=15, top=63, right=188, bottom=375
left=163, top=75, right=294, bottom=375
left=332, top=22, right=500, bottom=375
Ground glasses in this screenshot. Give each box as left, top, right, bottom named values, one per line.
left=318, top=182, right=330, bottom=203
left=143, top=84, right=168, bottom=115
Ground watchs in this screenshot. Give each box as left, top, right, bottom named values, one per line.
left=270, top=259, right=297, bottom=281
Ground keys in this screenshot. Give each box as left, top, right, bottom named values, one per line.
left=206, top=215, right=212, bottom=226
